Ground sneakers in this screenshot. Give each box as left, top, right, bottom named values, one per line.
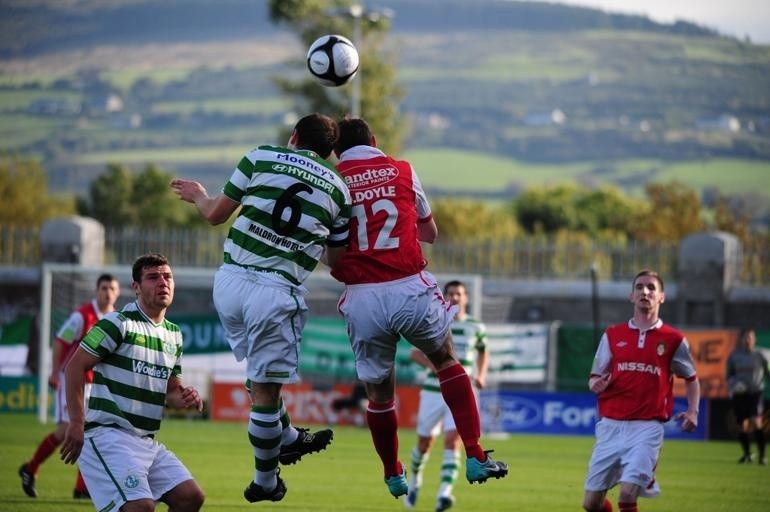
left=73, top=488, right=91, bottom=497
left=759, top=459, right=766, bottom=465
left=435, top=494, right=454, bottom=510
left=384, top=461, right=408, bottom=499
left=466, top=450, right=508, bottom=484
left=739, top=454, right=751, bottom=462
left=19, top=464, right=38, bottom=498
left=279, top=427, right=333, bottom=465
left=244, top=467, right=287, bottom=502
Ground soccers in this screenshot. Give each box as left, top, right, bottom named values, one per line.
left=305, top=35, right=358, bottom=87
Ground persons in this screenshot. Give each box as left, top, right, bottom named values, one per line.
left=60, top=250, right=206, bottom=511
left=403, top=279, right=488, bottom=511
left=17, top=273, right=121, bottom=499
left=321, top=117, right=510, bottom=497
left=167, top=109, right=353, bottom=501
left=578, top=269, right=701, bottom=512
left=724, top=327, right=770, bottom=464
